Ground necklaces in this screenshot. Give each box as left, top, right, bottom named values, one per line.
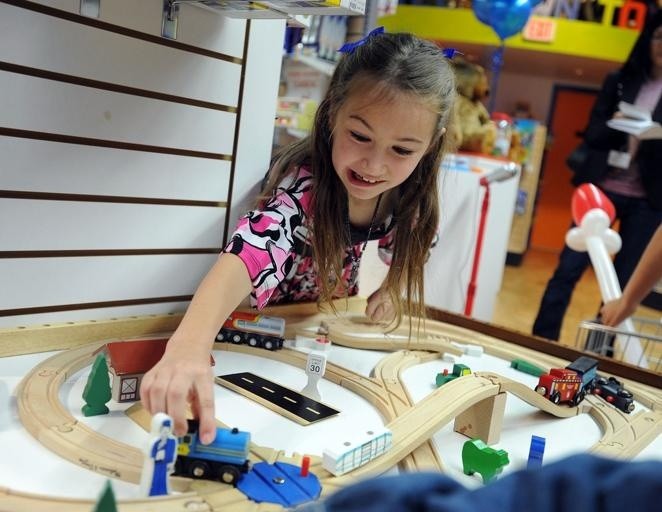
left=343, top=192, right=384, bottom=288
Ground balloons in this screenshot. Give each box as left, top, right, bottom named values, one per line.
left=471, top=0, right=541, bottom=43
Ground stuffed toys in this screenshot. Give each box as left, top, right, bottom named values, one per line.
left=441, top=53, right=499, bottom=155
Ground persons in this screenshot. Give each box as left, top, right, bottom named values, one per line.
left=530, top=5, right=662, bottom=344
left=598, top=221, right=662, bottom=335
left=137, top=21, right=459, bottom=449
